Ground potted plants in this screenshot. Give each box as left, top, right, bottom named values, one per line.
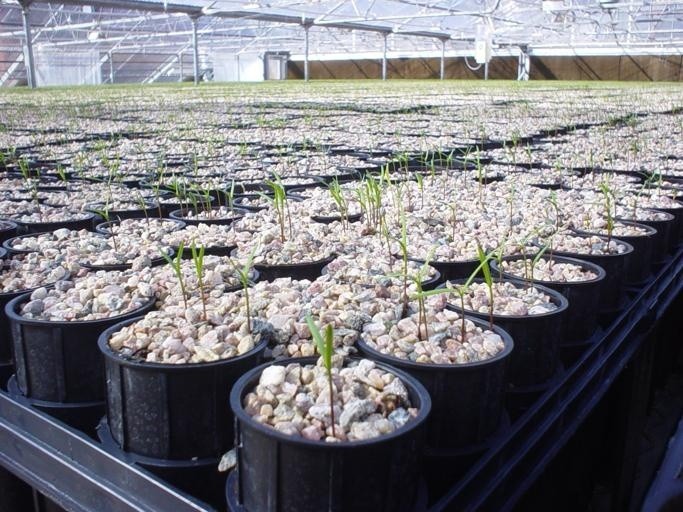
left=1, top=152, right=683, bottom=511
